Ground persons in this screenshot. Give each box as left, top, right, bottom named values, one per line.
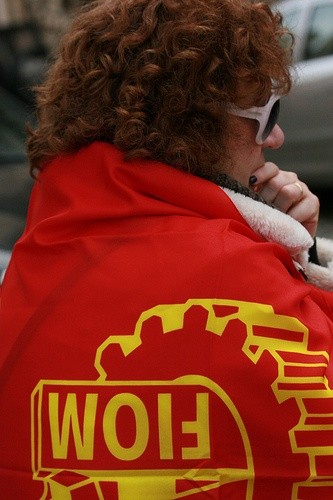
left=0, top=0, right=333, bottom=500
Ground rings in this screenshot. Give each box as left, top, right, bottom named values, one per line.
left=293, top=181, right=307, bottom=199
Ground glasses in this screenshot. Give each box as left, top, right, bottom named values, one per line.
left=225, top=92, right=282, bottom=145
